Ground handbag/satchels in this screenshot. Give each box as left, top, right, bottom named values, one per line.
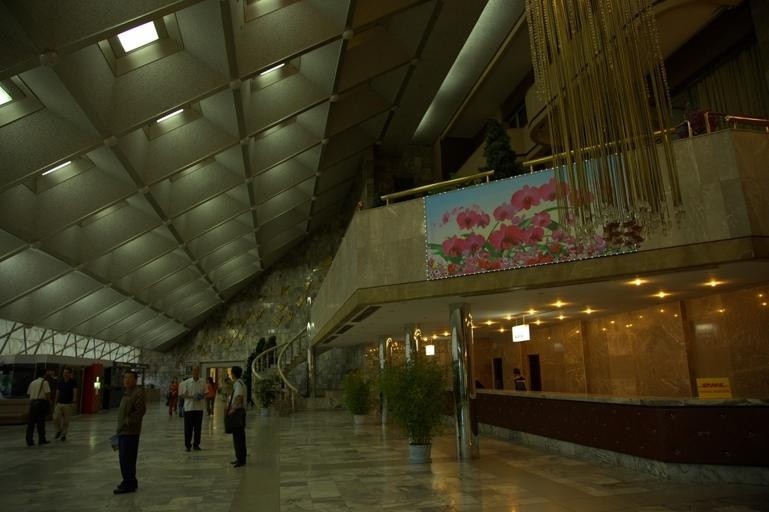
left=223, top=416, right=233, bottom=434
left=31, top=401, right=49, bottom=416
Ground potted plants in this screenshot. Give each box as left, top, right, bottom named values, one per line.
left=253, top=378, right=275, bottom=416
left=339, top=358, right=449, bottom=464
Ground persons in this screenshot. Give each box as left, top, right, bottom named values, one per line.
left=52, top=367, right=79, bottom=442
left=177, top=366, right=209, bottom=453
left=166, top=335, right=277, bottom=418
left=223, top=365, right=249, bottom=467
left=112, top=370, right=146, bottom=494
left=26, top=372, right=53, bottom=446
left=510, top=368, right=529, bottom=391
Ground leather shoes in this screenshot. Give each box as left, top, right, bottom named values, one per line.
left=61, top=436, right=64, bottom=441
left=192, top=447, right=200, bottom=450
left=26, top=439, right=33, bottom=445
left=55, top=431, right=61, bottom=438
left=233, top=464, right=241, bottom=467
left=230, top=461, right=236, bottom=464
left=116, top=482, right=138, bottom=488
left=184, top=447, right=190, bottom=451
left=112, top=488, right=135, bottom=494
left=39, top=440, right=49, bottom=444
